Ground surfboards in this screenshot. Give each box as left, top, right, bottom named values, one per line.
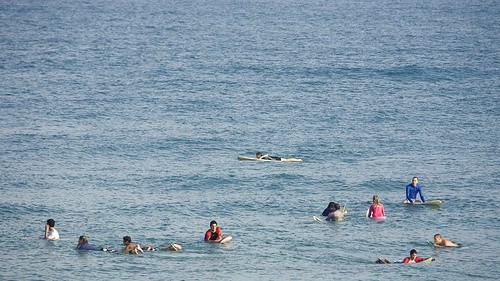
left=220, top=236, right=232, bottom=243
left=404, top=200, right=441, bottom=206
left=238, top=156, right=280, bottom=162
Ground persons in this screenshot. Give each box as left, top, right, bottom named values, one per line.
left=44, top=219, right=59, bottom=240
left=321, top=202, right=336, bottom=216
left=320, top=203, right=344, bottom=222
left=75, top=235, right=120, bottom=254
left=434, top=234, right=459, bottom=247
left=368, top=196, right=385, bottom=222
left=406, top=177, right=425, bottom=205
left=204, top=220, right=222, bottom=242
left=122, top=235, right=143, bottom=254
left=375, top=249, right=436, bottom=264
left=255, top=152, right=303, bottom=162
left=341, top=206, right=348, bottom=217
left=141, top=243, right=181, bottom=251
